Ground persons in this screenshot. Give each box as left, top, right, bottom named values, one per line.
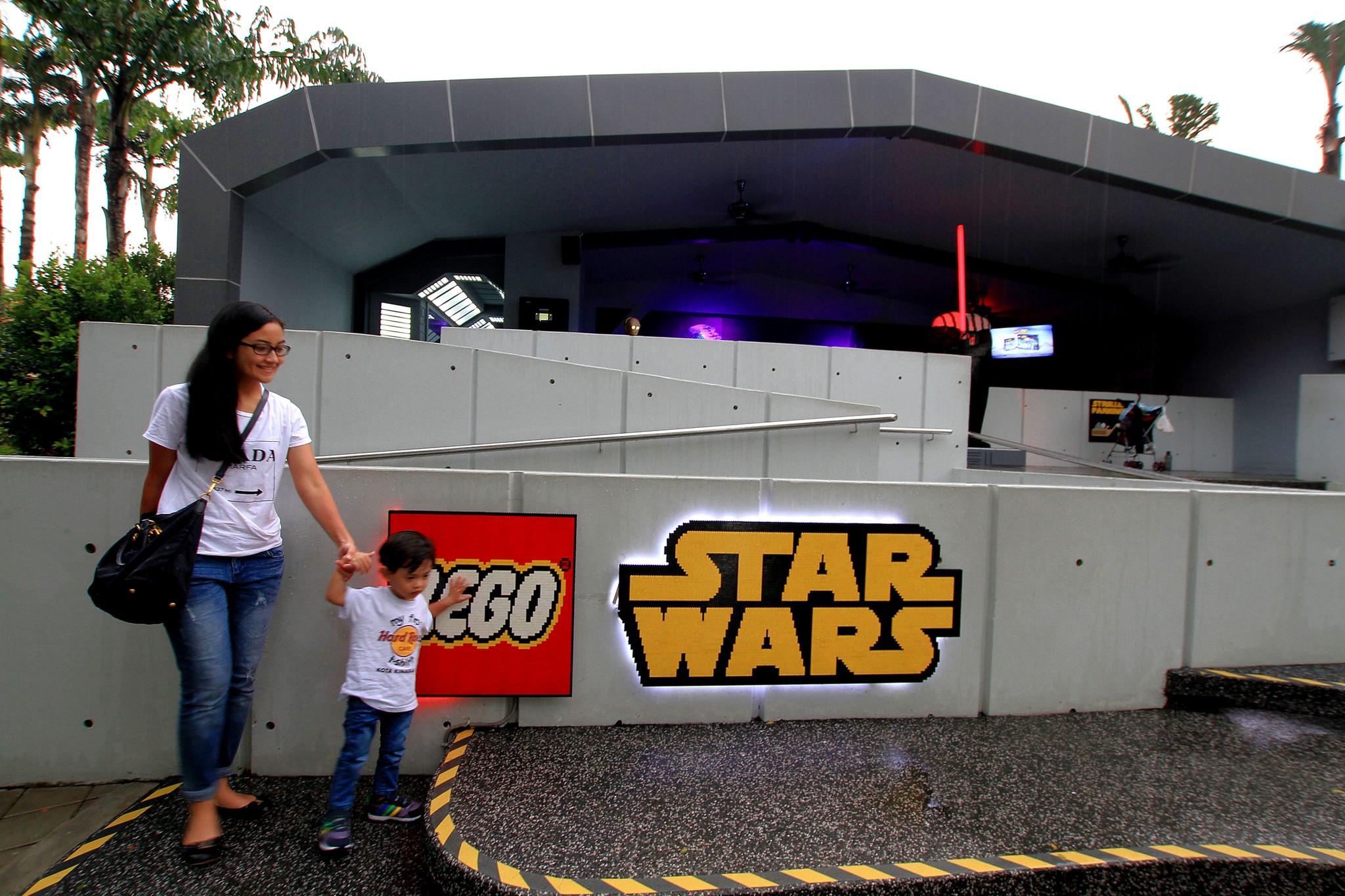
left=316, top=528, right=474, bottom=855
left=138, top=299, right=363, bottom=874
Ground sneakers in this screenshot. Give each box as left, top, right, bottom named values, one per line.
left=316, top=815, right=353, bottom=853
left=369, top=794, right=424, bottom=823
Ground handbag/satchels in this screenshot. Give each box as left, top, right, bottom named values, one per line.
left=87, top=491, right=214, bottom=625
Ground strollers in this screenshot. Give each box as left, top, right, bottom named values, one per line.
left=1102, top=390, right=1171, bottom=472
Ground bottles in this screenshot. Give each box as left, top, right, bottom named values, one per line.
left=1164, top=451, right=1173, bottom=471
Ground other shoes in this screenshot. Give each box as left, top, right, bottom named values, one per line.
left=218, top=793, right=275, bottom=819
left=182, top=823, right=225, bottom=867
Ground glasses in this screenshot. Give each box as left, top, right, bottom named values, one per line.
left=241, top=340, right=294, bottom=361
